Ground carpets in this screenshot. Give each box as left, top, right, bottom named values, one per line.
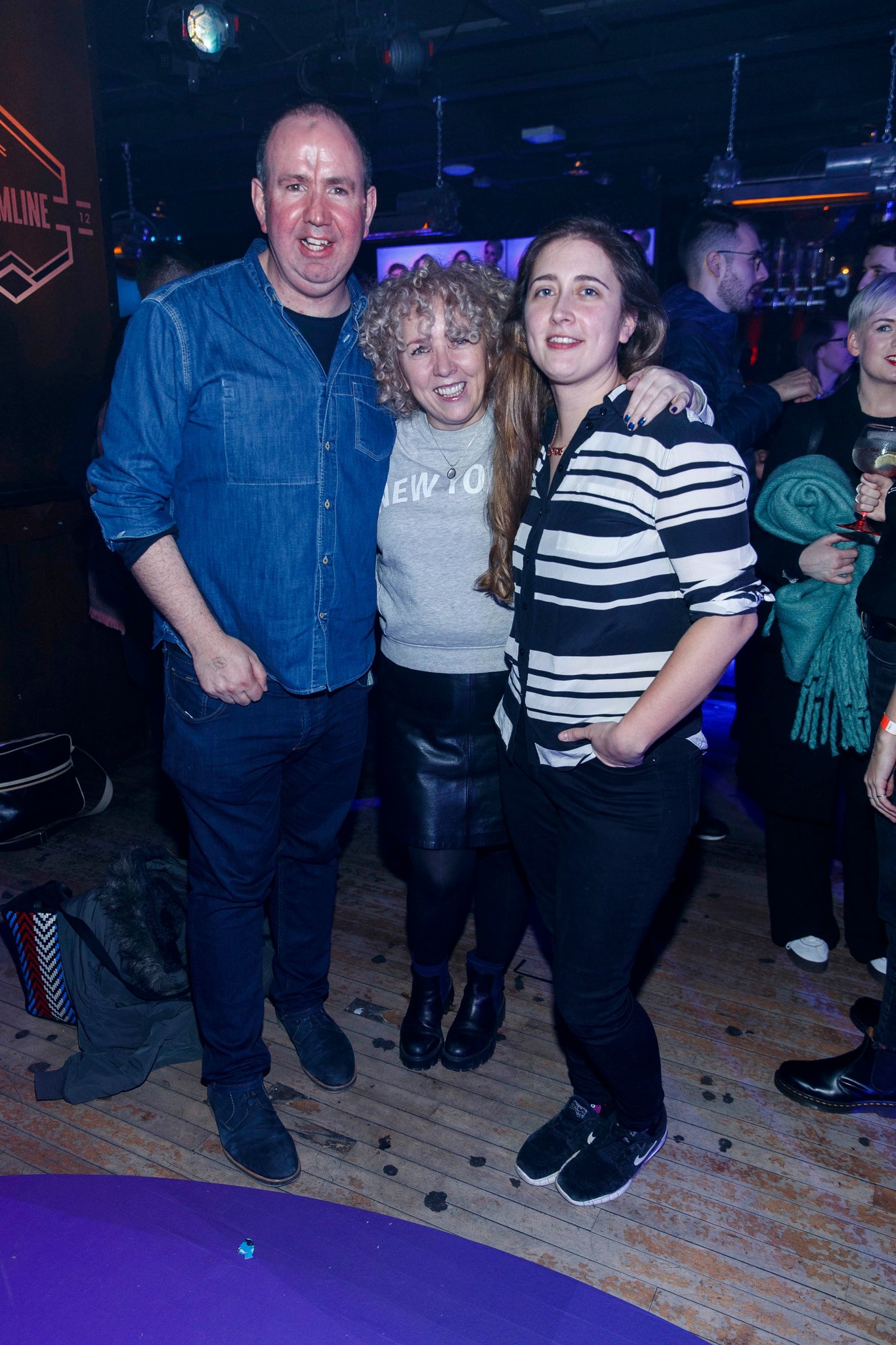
left=0, top=1175, right=711, bottom=1345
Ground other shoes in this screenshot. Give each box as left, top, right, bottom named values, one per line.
left=786, top=934, right=829, bottom=974
left=693, top=810, right=728, bottom=840
left=867, top=956, right=888, bottom=982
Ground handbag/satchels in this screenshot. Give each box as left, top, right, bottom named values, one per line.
left=0, top=732, right=113, bottom=845
left=0, top=880, right=78, bottom=1027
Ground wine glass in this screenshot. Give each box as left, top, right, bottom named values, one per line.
left=835, top=424, right=896, bottom=536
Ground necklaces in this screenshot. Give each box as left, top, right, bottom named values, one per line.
left=428, top=412, right=486, bottom=478
left=547, top=374, right=623, bottom=456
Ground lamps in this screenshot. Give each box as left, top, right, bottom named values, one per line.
left=702, top=30, right=896, bottom=219
left=143, top=0, right=238, bottom=77
left=111, top=143, right=184, bottom=259
left=365, top=95, right=463, bottom=241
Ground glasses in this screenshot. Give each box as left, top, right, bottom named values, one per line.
left=715, top=250, right=763, bottom=271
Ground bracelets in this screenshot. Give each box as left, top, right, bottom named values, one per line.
left=880, top=712, right=896, bottom=736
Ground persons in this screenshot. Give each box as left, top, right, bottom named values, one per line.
left=648, top=205, right=823, bottom=844
left=478, top=220, right=764, bottom=1205
left=389, top=263, right=408, bottom=278
left=732, top=221, right=896, bottom=1117
left=88, top=102, right=397, bottom=1184
left=411, top=254, right=440, bottom=271
left=357, top=261, right=716, bottom=1073
left=484, top=241, right=503, bottom=264
left=452, top=250, right=472, bottom=264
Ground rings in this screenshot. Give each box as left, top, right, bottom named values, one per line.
left=869, top=797, right=873, bottom=799
left=855, top=494, right=860, bottom=504
left=877, top=795, right=886, bottom=798
left=856, top=483, right=863, bottom=493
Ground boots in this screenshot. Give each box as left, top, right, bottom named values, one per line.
left=442, top=949, right=508, bottom=1071
left=398, top=961, right=455, bottom=1074
left=774, top=1026, right=896, bottom=1120
left=850, top=997, right=884, bottom=1036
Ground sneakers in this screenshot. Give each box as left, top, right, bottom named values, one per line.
left=514, top=1092, right=619, bottom=1186
left=276, top=1006, right=356, bottom=1091
left=553, top=1105, right=669, bottom=1204
left=206, top=1078, right=301, bottom=1187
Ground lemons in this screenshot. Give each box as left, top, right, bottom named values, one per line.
left=873, top=453, right=896, bottom=468
